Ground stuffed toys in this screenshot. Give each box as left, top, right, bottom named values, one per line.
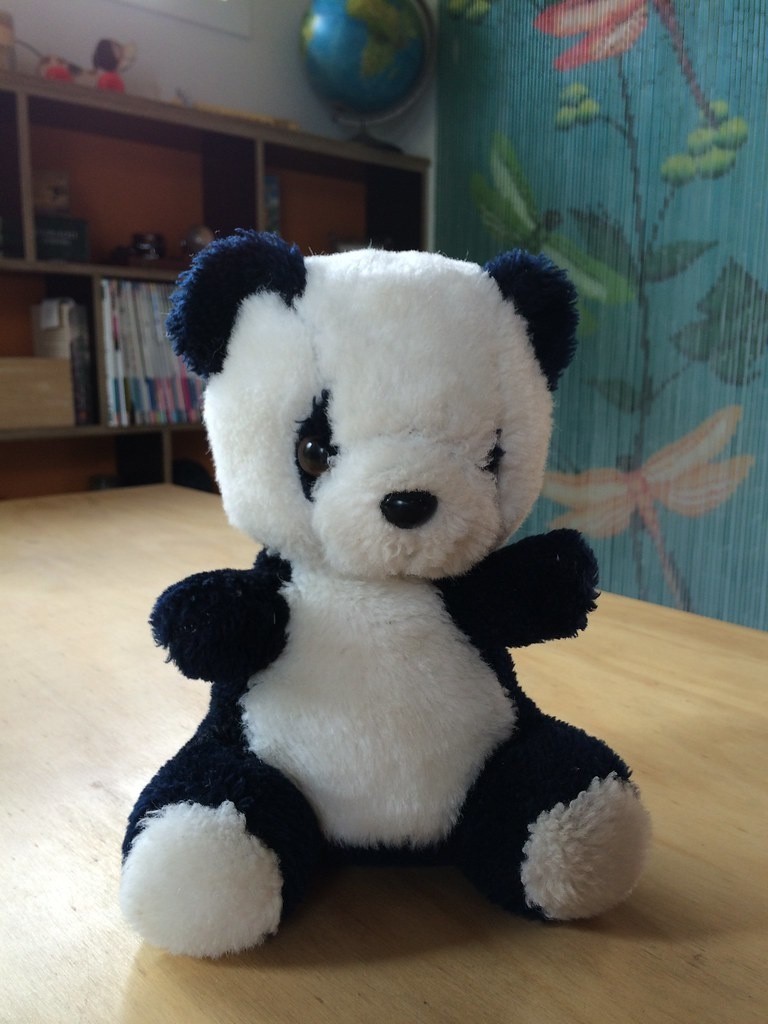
left=122, top=226, right=656, bottom=962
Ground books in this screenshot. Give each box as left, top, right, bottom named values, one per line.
left=28, top=278, right=207, bottom=429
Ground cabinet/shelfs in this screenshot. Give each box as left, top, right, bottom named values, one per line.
left=0, top=70, right=429, bottom=500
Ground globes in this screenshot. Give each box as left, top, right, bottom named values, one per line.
left=301, top=0, right=437, bottom=155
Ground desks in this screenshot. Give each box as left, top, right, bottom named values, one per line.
left=0, top=483, right=768, bottom=1023
